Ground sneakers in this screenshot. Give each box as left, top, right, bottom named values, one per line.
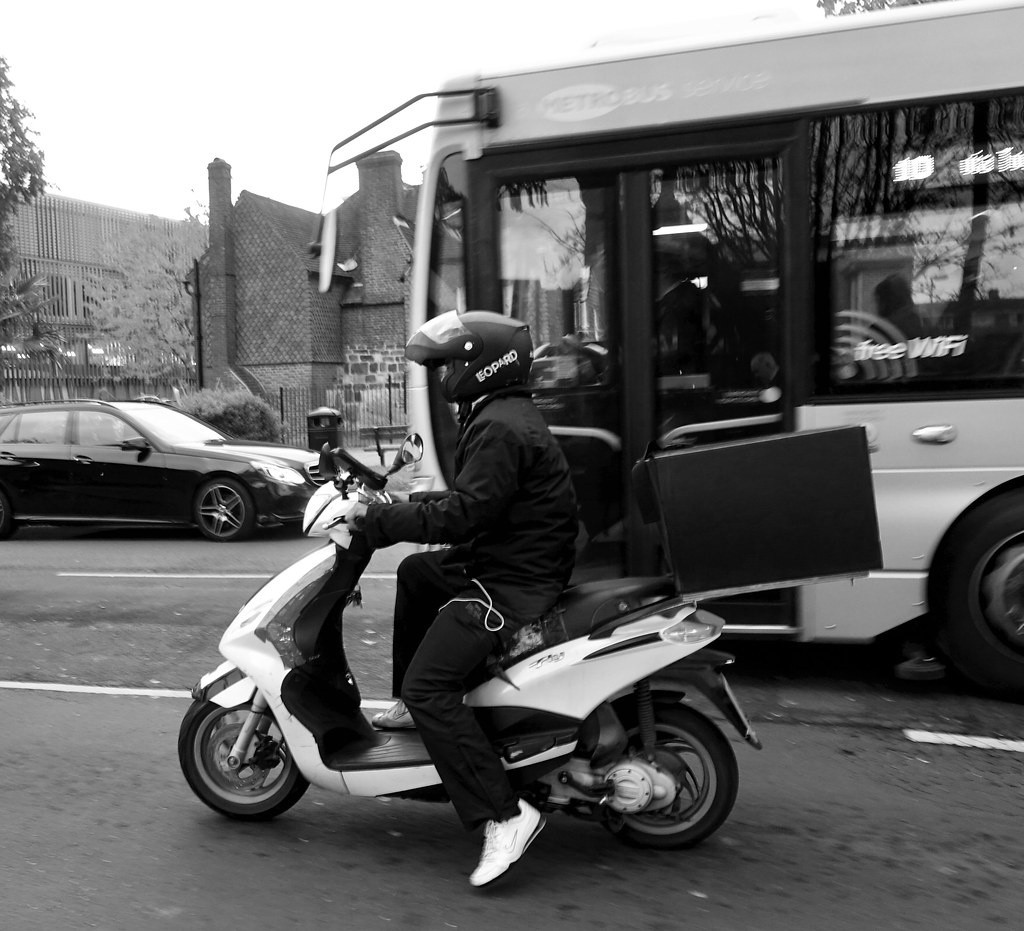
left=469, top=796, right=545, bottom=886
left=372, top=699, right=417, bottom=727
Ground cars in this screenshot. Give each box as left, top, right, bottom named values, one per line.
left=0, top=397, right=328, bottom=542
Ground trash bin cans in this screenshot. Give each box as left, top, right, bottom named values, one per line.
left=305, top=406, right=343, bottom=451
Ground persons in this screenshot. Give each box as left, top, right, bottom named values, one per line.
left=322, top=307, right=580, bottom=892
left=649, top=250, right=941, bottom=384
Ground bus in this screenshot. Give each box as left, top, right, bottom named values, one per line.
left=305, top=0, right=1024, bottom=712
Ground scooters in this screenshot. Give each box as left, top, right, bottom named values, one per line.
left=177, top=432, right=763, bottom=853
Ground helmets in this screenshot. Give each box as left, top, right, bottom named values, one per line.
left=405, top=311, right=535, bottom=402
